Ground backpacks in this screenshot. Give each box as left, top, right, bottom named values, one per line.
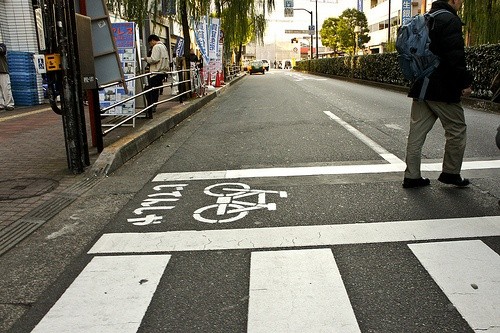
left=396, top=7, right=453, bottom=98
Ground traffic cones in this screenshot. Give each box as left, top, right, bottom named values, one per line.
left=219, top=70, right=226, bottom=85
left=214, top=71, right=221, bottom=87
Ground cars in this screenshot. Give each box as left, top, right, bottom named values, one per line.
left=242, top=57, right=270, bottom=74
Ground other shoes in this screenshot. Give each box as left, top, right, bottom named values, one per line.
left=402, top=174, right=430, bottom=188
left=438, top=172, right=471, bottom=186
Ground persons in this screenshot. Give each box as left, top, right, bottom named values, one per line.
left=274, top=60, right=283, bottom=69
left=143, top=35, right=170, bottom=119
left=401, top=0, right=474, bottom=189
